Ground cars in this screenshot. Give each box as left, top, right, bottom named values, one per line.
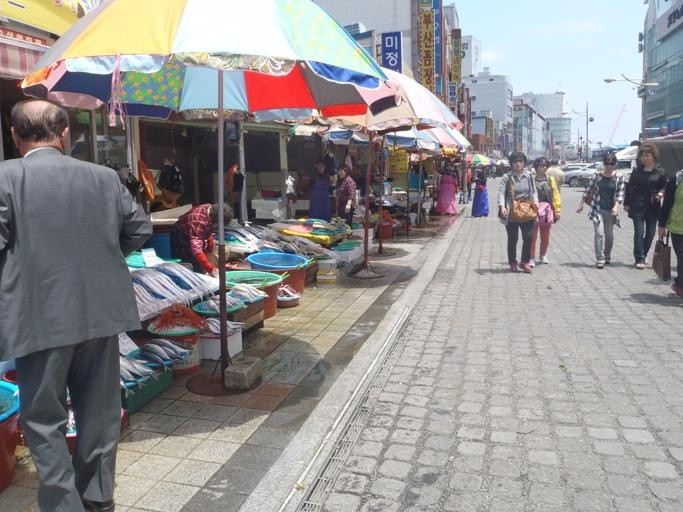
left=552, top=161, right=603, bottom=188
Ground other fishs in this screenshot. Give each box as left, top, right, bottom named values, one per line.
left=225, top=225, right=323, bottom=259
left=115, top=262, right=270, bottom=397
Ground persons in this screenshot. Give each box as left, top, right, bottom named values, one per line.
left=0, top=97, right=154, bottom=512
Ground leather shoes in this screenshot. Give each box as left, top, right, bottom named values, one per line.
left=81, top=497, right=114, bottom=512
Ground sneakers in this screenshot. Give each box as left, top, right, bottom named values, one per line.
left=597, top=263, right=602, bottom=269
left=671, top=283, right=678, bottom=291
left=604, top=258, right=609, bottom=264
left=635, top=262, right=644, bottom=269
left=510, top=254, right=548, bottom=273
left=674, top=289, right=682, bottom=297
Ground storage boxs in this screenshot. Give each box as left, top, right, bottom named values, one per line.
left=317, top=228, right=374, bottom=270
left=314, top=268, right=341, bottom=287
left=252, top=197, right=310, bottom=219
left=199, top=323, right=244, bottom=360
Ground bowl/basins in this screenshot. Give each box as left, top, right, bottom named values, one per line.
left=245, top=252, right=310, bottom=271
left=0, top=380, right=22, bottom=423
left=224, top=270, right=284, bottom=287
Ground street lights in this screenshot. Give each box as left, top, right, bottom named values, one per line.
left=602, top=72, right=650, bottom=140
left=560, top=102, right=593, bottom=151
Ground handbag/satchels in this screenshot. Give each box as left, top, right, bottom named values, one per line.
left=510, top=200, right=538, bottom=223
left=585, top=172, right=598, bottom=206
left=652, top=238, right=670, bottom=281
left=536, top=202, right=553, bottom=227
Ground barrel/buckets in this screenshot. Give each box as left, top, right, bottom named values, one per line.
left=380, top=222, right=393, bottom=239
left=250, top=269, right=305, bottom=295
left=225, top=281, right=280, bottom=319
left=148, top=317, right=202, bottom=375
left=1, top=381, right=21, bottom=493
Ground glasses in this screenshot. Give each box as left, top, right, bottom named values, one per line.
left=604, top=161, right=615, bottom=165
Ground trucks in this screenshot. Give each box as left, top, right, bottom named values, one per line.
left=598, top=138, right=682, bottom=194
